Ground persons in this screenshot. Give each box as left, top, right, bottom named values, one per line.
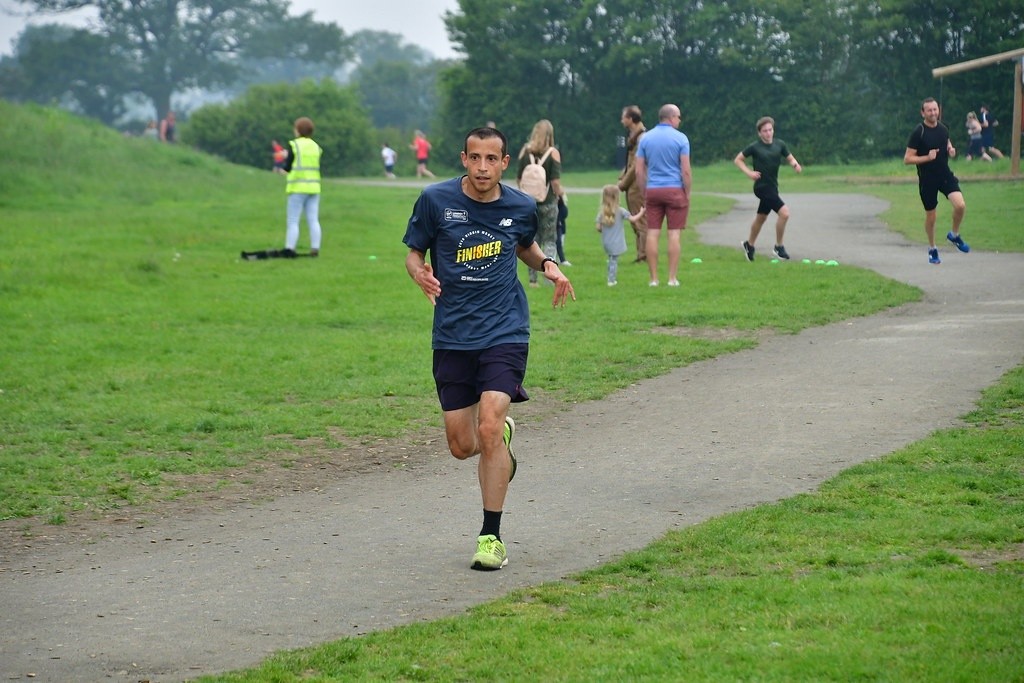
left=409, top=130, right=434, bottom=177
left=402, top=127, right=577, bottom=568
left=733, top=117, right=802, bottom=261
left=280, top=117, right=324, bottom=255
left=556, top=186, right=571, bottom=264
left=979, top=104, right=1004, bottom=161
left=160, top=110, right=176, bottom=142
left=382, top=143, right=397, bottom=177
left=965, top=111, right=991, bottom=161
left=904, top=98, right=969, bottom=263
left=618, top=106, right=647, bottom=262
left=595, top=184, right=646, bottom=285
left=273, top=140, right=286, bottom=174
left=635, top=104, right=691, bottom=285
left=144, top=121, right=157, bottom=139
left=516, top=119, right=564, bottom=287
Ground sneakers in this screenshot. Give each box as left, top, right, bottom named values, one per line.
left=501, top=416, right=517, bottom=483
left=471, top=534, right=508, bottom=571
left=928, top=246, right=941, bottom=265
left=741, top=241, right=755, bottom=261
left=773, top=244, right=789, bottom=259
left=947, top=232, right=970, bottom=253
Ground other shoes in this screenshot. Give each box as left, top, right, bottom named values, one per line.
left=608, top=281, right=617, bottom=286
left=668, top=280, right=679, bottom=286
left=560, top=260, right=571, bottom=266
left=530, top=282, right=539, bottom=287
left=636, top=256, right=647, bottom=262
left=312, top=249, right=318, bottom=256
left=650, top=281, right=658, bottom=286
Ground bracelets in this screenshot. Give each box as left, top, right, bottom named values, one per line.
left=541, top=257, right=558, bottom=272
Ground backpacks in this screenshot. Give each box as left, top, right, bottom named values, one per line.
left=520, top=146, right=553, bottom=202
